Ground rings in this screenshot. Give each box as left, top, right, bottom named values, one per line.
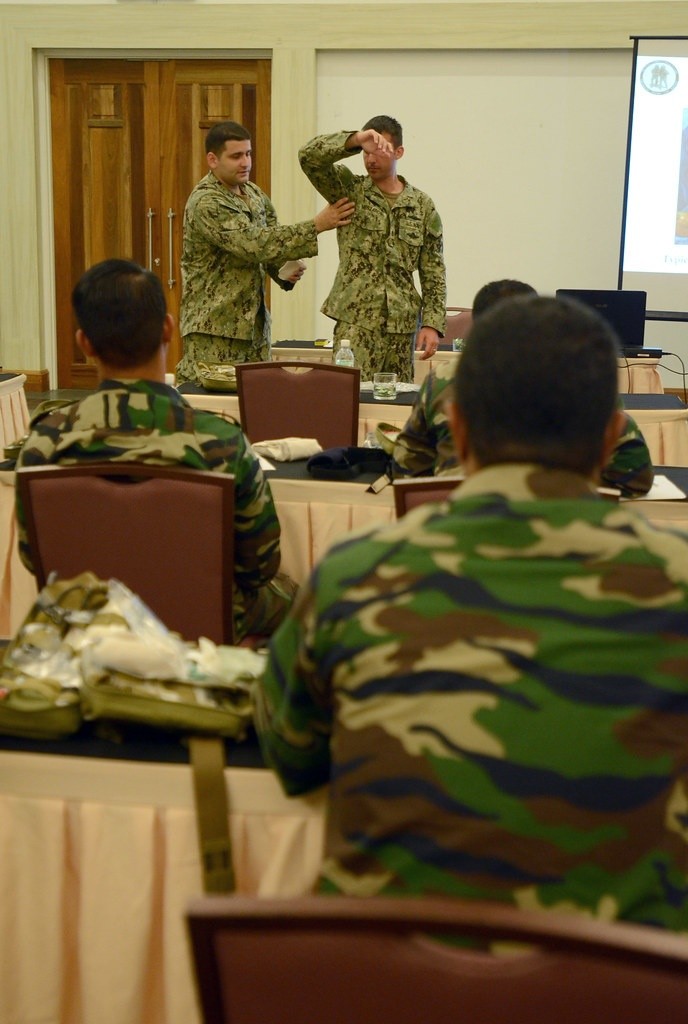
left=433, top=349, right=437, bottom=351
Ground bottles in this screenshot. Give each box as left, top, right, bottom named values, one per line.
left=164, top=373, right=179, bottom=393
left=335, top=339, right=355, bottom=367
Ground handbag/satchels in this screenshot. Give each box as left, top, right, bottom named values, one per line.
left=305, top=445, right=392, bottom=481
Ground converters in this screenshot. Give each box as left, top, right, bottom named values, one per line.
left=624, top=347, right=663, bottom=358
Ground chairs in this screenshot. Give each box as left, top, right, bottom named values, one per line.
left=18, top=463, right=236, bottom=643
left=186, top=890, right=688, bottom=1024
left=436, top=307, right=474, bottom=345
left=235, top=361, right=361, bottom=451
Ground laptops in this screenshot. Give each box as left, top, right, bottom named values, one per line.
left=393, top=475, right=464, bottom=519
left=556, top=289, right=647, bottom=347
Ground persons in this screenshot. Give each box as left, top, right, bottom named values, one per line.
left=14, top=257, right=301, bottom=647
left=297, top=116, right=448, bottom=383
left=251, top=279, right=688, bottom=957
left=175, top=120, right=355, bottom=384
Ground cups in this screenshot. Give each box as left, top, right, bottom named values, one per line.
left=374, top=373, right=397, bottom=400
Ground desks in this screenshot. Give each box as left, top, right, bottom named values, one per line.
left=270, top=339, right=664, bottom=396
left=176, top=381, right=688, bottom=468
left=0, top=719, right=329, bottom=1024
left=1, top=448, right=688, bottom=642
